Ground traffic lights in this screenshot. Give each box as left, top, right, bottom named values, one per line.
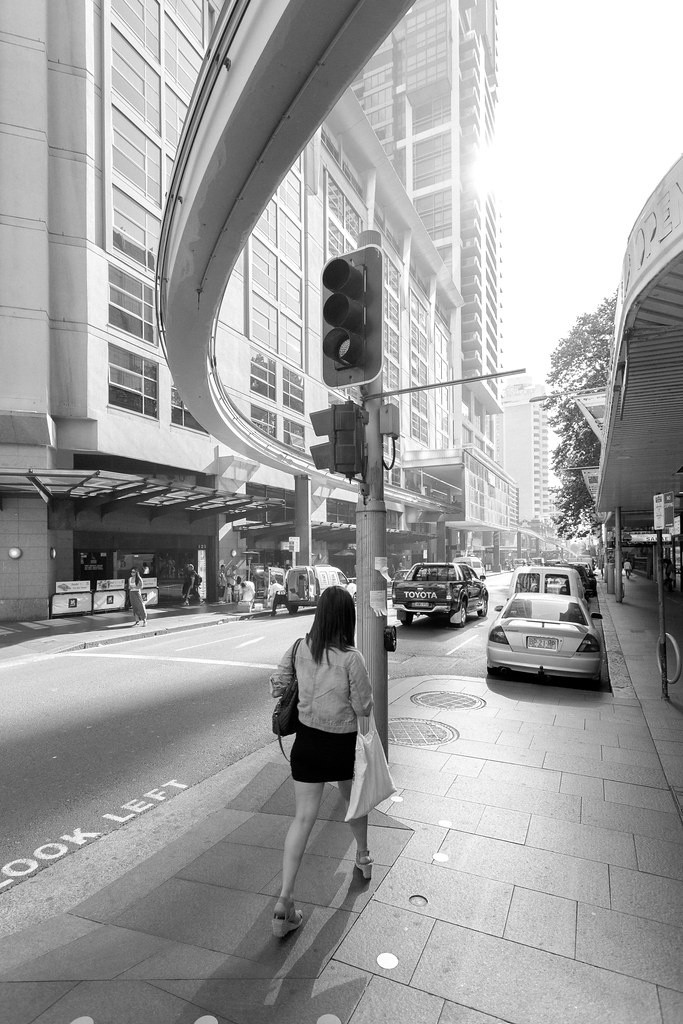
left=308, top=399, right=371, bottom=477
left=320, top=243, right=385, bottom=387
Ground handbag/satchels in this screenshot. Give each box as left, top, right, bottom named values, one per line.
left=272, top=638, right=303, bottom=736
left=344, top=709, right=397, bottom=823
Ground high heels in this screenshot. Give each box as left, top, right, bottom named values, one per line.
left=355, top=850, right=373, bottom=879
left=272, top=896, right=303, bottom=937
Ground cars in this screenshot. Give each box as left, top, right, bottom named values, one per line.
left=545, top=557, right=599, bottom=598
left=486, top=592, right=607, bottom=688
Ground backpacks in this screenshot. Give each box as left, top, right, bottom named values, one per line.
left=193, top=573, right=202, bottom=589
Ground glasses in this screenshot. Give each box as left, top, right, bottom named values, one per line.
left=131, top=571, right=136, bottom=573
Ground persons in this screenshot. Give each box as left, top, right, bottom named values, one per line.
left=389, top=562, right=405, bottom=579
left=270, top=586, right=374, bottom=939
left=143, top=559, right=254, bottom=608
left=665, top=559, right=674, bottom=592
left=502, top=557, right=538, bottom=568
left=129, top=568, right=148, bottom=629
left=623, top=559, right=632, bottom=579
left=267, top=580, right=295, bottom=616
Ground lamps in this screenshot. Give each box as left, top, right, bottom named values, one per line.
left=9, top=546, right=22, bottom=560
left=51, top=547, right=57, bottom=559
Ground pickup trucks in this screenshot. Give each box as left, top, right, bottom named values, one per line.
left=390, top=561, right=489, bottom=627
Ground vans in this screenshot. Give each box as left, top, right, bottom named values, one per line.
left=450, top=555, right=487, bottom=582
left=273, top=564, right=356, bottom=613
left=509, top=565, right=593, bottom=617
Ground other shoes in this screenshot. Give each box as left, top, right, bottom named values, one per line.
left=183, top=602, right=190, bottom=606
left=132, top=621, right=140, bottom=627
left=200, top=600, right=206, bottom=605
left=217, top=601, right=226, bottom=604
left=143, top=622, right=147, bottom=627
